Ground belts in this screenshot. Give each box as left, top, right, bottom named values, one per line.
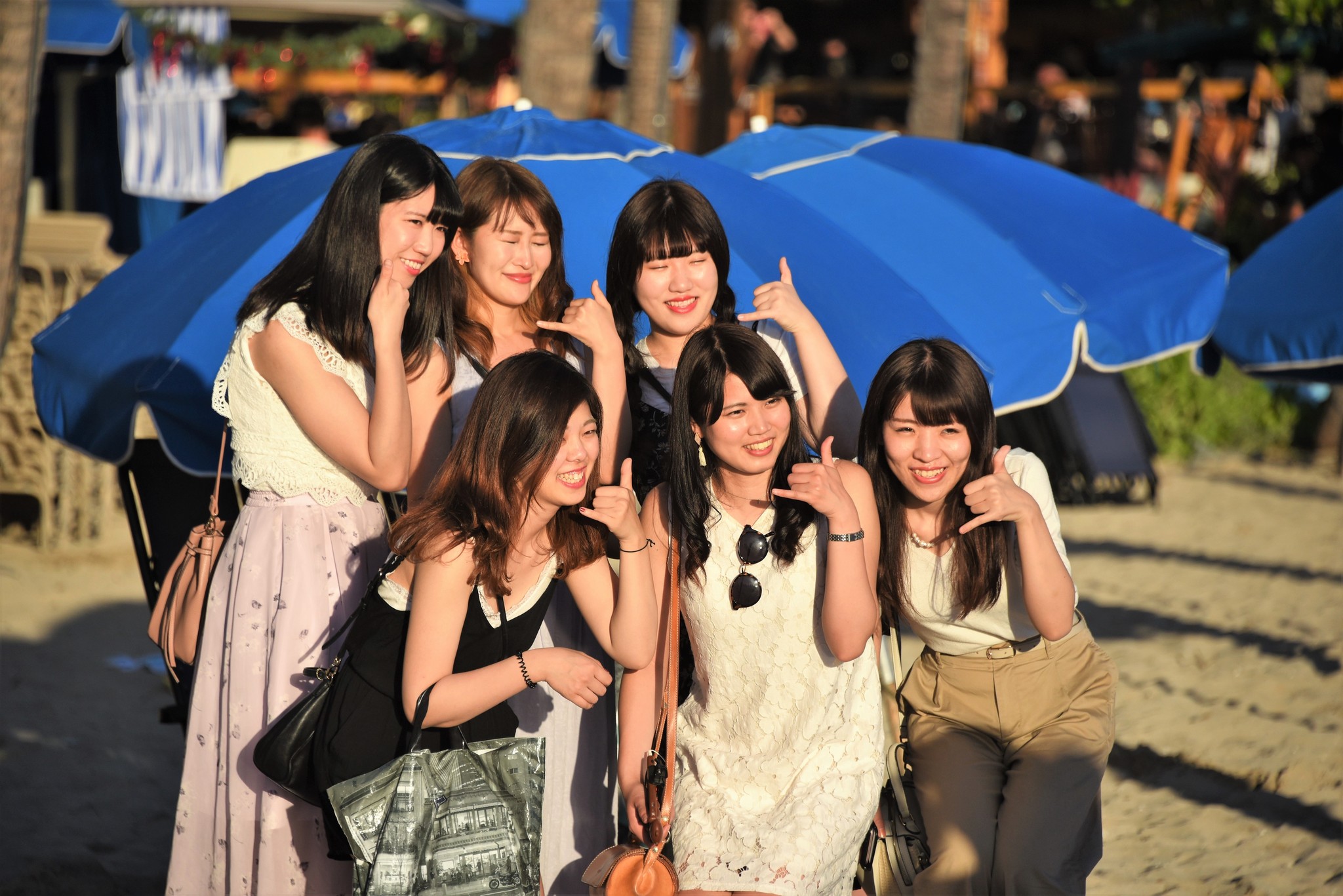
left=958, top=618, right=1087, bottom=660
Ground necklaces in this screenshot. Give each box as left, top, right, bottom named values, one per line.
left=904, top=510, right=959, bottom=551
left=715, top=468, right=773, bottom=506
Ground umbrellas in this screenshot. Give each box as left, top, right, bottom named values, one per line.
left=26, top=94, right=995, bottom=494
left=1184, top=177, right=1343, bottom=403
left=712, top=110, right=1232, bottom=466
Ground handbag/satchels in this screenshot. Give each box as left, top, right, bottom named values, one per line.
left=579, top=841, right=679, bottom=896
left=324, top=682, right=545, bottom=896
left=250, top=646, right=333, bottom=807
left=856, top=786, right=928, bottom=896
left=148, top=518, right=240, bottom=664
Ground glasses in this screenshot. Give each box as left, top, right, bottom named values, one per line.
left=729, top=524, right=770, bottom=611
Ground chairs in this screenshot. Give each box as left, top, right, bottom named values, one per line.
left=0, top=179, right=124, bottom=552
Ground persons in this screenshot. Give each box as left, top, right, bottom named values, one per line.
left=853, top=335, right=1120, bottom=896
left=615, top=319, right=897, bottom=896
left=602, top=175, right=865, bottom=530
left=311, top=350, right=667, bottom=896
left=161, top=129, right=470, bottom=896
left=954, top=37, right=1343, bottom=263
left=215, top=77, right=414, bottom=149
left=418, top=155, right=641, bottom=896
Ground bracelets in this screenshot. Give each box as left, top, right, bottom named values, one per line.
left=515, top=650, right=540, bottom=691
left=618, top=537, right=657, bottom=554
left=826, top=528, right=865, bottom=544
